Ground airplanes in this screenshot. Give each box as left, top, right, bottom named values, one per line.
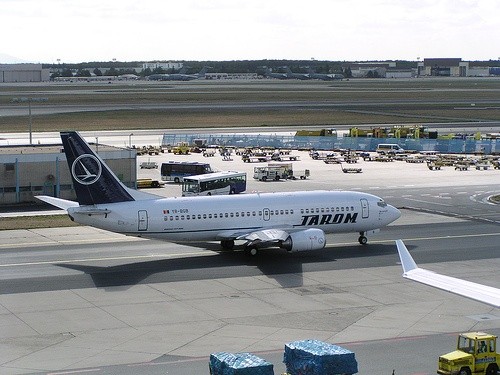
left=34, top=131, right=402, bottom=258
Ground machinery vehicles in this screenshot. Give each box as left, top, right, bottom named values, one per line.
left=173, top=141, right=192, bottom=155
left=436, top=330, right=500, bottom=375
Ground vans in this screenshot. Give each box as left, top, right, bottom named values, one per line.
left=376, top=144, right=405, bottom=154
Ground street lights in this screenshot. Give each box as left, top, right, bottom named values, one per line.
left=128, top=132, right=134, bottom=189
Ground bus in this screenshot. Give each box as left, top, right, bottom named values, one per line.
left=160, top=161, right=213, bottom=184
left=181, top=170, right=246, bottom=197
left=252, top=165, right=293, bottom=181
